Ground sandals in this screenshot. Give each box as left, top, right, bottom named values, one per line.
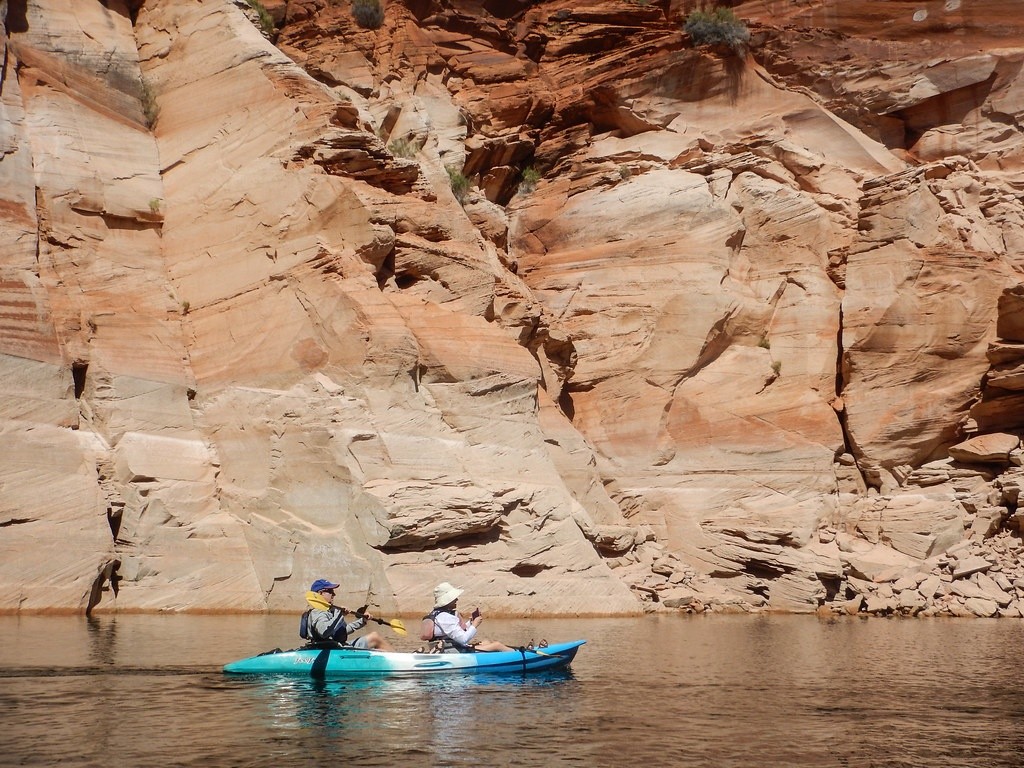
left=412, top=647, right=424, bottom=653
left=539, top=639, right=548, bottom=648
left=429, top=640, right=444, bottom=654
left=527, top=639, right=534, bottom=649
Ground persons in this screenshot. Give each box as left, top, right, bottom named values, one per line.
left=428, top=582, right=548, bottom=654
left=306, top=579, right=395, bottom=652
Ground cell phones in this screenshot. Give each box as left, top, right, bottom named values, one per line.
left=472, top=608, right=480, bottom=621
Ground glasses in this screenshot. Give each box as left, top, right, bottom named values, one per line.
left=320, top=589, right=334, bottom=594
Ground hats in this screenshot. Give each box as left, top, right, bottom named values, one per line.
left=311, top=579, right=340, bottom=592
left=432, top=583, right=464, bottom=609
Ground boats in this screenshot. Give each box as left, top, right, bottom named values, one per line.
left=221, top=639, right=588, bottom=685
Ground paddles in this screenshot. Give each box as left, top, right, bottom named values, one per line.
left=304, top=590, right=407, bottom=636
left=505, top=643, right=561, bottom=658
